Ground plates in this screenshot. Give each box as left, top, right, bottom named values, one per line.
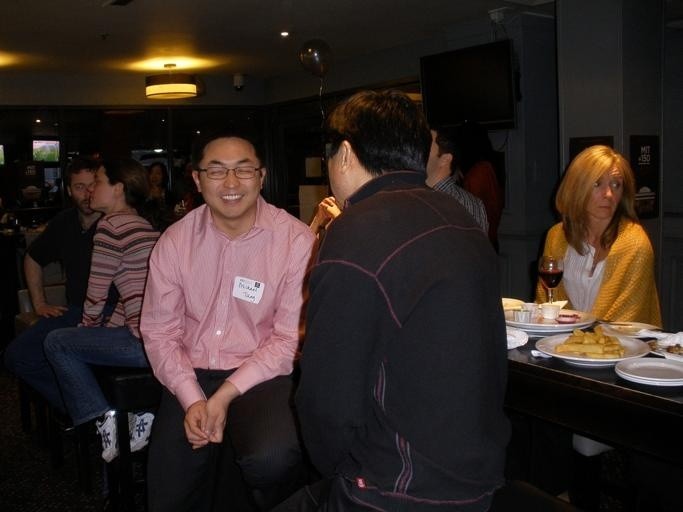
left=535, top=333, right=651, bottom=367
left=615, top=357, right=683, bottom=388
left=501, top=297, right=527, bottom=312
left=596, top=322, right=666, bottom=339
left=502, top=307, right=598, bottom=331
left=505, top=326, right=528, bottom=349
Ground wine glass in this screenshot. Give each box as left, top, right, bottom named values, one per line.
left=538, top=255, right=564, bottom=303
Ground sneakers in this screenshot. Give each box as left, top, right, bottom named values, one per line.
left=129, top=412, right=154, bottom=453
left=96, top=411, right=119, bottom=462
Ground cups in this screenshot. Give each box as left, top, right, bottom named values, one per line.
left=542, top=304, right=559, bottom=319
left=522, top=302, right=538, bottom=318
left=511, top=309, right=531, bottom=323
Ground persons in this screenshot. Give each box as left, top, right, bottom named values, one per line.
left=0, top=156, right=121, bottom=443
left=413, top=115, right=491, bottom=239
left=270, top=88, right=513, bottom=512
left=534, top=142, right=663, bottom=330
left=302, top=194, right=344, bottom=239
left=41, top=151, right=162, bottom=464
left=2, top=128, right=209, bottom=362
left=138, top=114, right=325, bottom=511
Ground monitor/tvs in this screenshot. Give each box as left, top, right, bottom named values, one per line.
left=0, top=144, right=6, bottom=165
left=29, top=135, right=62, bottom=166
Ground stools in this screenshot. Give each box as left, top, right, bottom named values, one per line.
left=12, top=307, right=165, bottom=510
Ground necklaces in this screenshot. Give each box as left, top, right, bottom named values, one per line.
left=591, top=241, right=600, bottom=269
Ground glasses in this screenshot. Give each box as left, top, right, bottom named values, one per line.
left=198, top=166, right=264, bottom=180
